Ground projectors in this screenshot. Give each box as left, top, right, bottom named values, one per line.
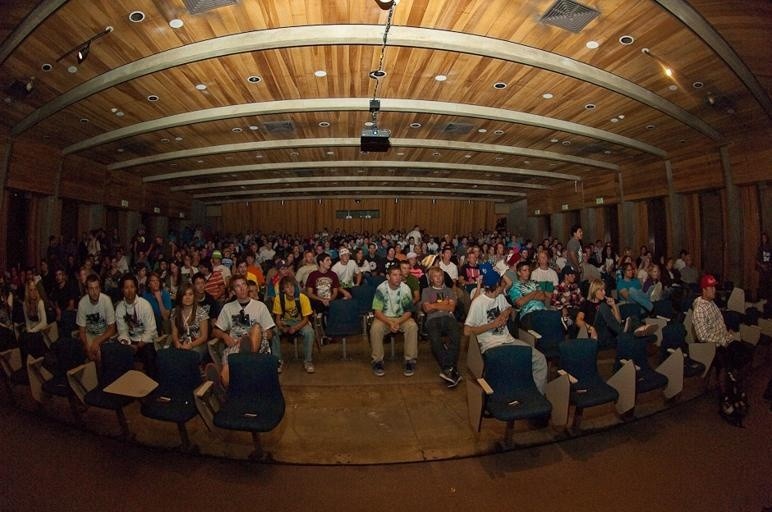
left=360, top=128, right=392, bottom=152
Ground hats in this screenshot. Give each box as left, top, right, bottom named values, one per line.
left=339, top=248, right=352, bottom=258
left=483, top=270, right=500, bottom=289
left=698, top=275, right=719, bottom=290
left=505, top=253, right=521, bottom=268
left=561, top=265, right=578, bottom=274
left=421, top=253, right=439, bottom=272
left=407, top=252, right=417, bottom=259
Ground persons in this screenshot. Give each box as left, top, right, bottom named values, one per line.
left=2, top=221, right=770, bottom=421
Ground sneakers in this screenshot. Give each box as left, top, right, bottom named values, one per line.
left=403, top=358, right=416, bottom=376
left=373, top=361, right=385, bottom=376
left=620, top=317, right=632, bottom=333
left=439, top=366, right=463, bottom=387
left=278, top=359, right=284, bottom=373
left=303, top=360, right=314, bottom=373
left=633, top=323, right=659, bottom=337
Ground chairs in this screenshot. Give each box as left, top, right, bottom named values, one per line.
left=291, top=275, right=388, bottom=362
left=0, top=332, right=287, bottom=464
